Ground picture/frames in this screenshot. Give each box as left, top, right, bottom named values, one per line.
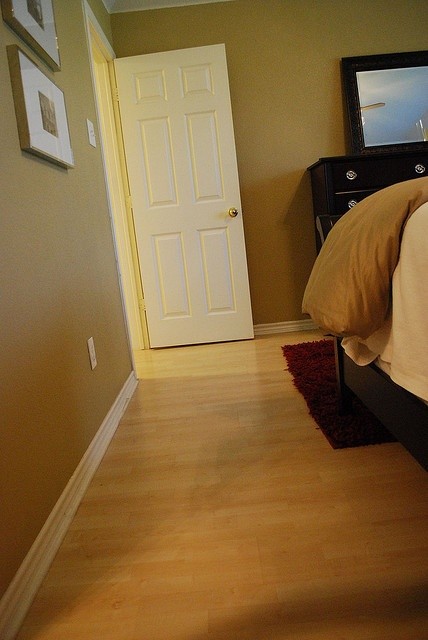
left=5, top=44, right=77, bottom=170
left=0, top=0, right=62, bottom=73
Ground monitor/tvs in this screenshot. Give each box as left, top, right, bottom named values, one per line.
left=338, top=48, right=428, bottom=152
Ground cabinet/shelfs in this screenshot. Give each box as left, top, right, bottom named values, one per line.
left=307, top=149, right=427, bottom=257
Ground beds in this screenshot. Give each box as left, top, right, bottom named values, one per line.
left=301, top=177, right=428, bottom=472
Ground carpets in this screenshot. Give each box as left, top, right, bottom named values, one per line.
left=280, top=339, right=398, bottom=449
